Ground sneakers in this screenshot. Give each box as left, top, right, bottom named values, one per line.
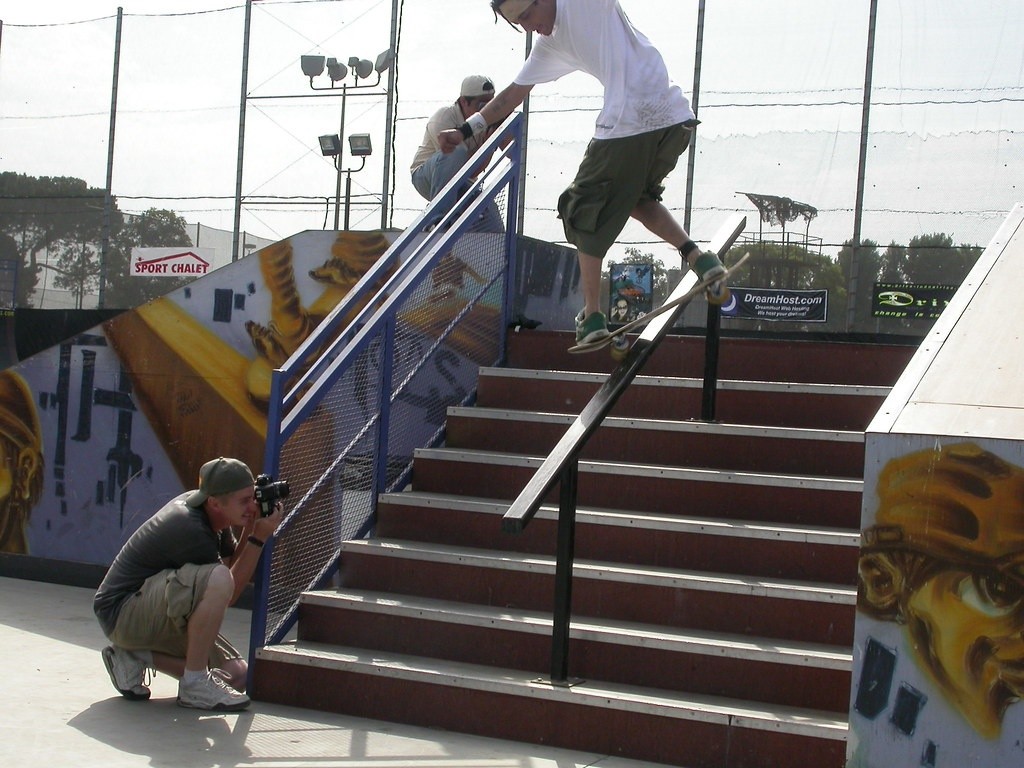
left=574, top=306, right=610, bottom=346
left=177, top=669, right=251, bottom=711
left=689, top=250, right=727, bottom=282
left=102, top=644, right=156, bottom=700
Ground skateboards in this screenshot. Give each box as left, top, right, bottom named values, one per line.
left=567, top=252, right=750, bottom=361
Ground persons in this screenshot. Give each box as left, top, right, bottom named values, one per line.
left=438, top=0, right=729, bottom=345
left=94, top=457, right=284, bottom=712
left=411, top=75, right=515, bottom=233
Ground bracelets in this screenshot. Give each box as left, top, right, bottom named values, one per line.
left=247, top=535, right=264, bottom=547
left=466, top=111, right=488, bottom=135
left=457, top=122, right=473, bottom=141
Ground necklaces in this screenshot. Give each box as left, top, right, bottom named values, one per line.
left=473, top=136, right=486, bottom=172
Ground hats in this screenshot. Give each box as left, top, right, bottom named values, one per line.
left=186, top=457, right=255, bottom=507
left=455, top=75, right=495, bottom=102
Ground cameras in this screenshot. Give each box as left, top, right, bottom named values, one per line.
left=254, top=474, right=289, bottom=518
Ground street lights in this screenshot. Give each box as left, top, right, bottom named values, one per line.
left=319, top=132, right=373, bottom=230
